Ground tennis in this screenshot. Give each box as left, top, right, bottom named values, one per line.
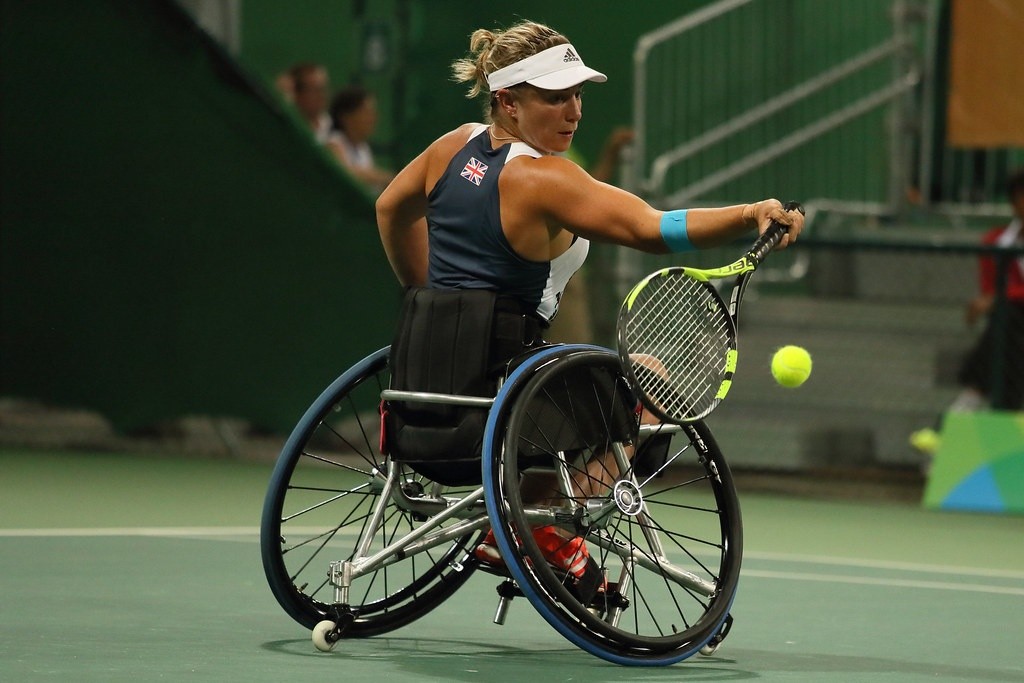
left=768, top=343, right=814, bottom=389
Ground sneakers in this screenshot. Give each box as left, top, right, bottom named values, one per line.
left=475, top=526, right=532, bottom=569
left=515, top=520, right=607, bottom=588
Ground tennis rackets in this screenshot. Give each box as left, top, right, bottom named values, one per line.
left=612, top=200, right=807, bottom=427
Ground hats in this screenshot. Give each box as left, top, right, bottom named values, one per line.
left=485, top=43, right=607, bottom=90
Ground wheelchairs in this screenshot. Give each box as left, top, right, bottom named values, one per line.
left=262, top=342, right=737, bottom=663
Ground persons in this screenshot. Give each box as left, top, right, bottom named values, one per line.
left=375, top=16, right=806, bottom=601
left=272, top=59, right=635, bottom=347
left=911, top=168, right=1024, bottom=477
